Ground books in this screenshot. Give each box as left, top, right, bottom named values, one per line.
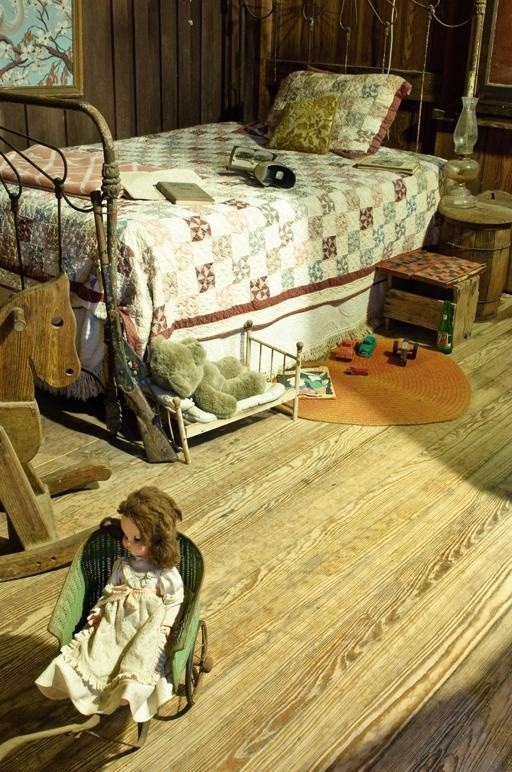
left=155, top=181, right=216, bottom=207
left=117, top=168, right=218, bottom=200
left=278, top=359, right=338, bottom=403
left=356, top=155, right=422, bottom=177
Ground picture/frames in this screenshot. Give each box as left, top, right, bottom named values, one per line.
left=0, top=0, right=85, bottom=98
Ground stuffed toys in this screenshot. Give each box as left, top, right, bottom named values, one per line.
left=356, top=334, right=377, bottom=359
left=149, top=331, right=267, bottom=421
left=334, top=336, right=356, bottom=361
left=390, top=337, right=421, bottom=368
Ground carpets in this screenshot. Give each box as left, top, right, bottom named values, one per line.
left=272, top=337, right=472, bottom=426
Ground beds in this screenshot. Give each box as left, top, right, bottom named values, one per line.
left=0, top=93, right=451, bottom=436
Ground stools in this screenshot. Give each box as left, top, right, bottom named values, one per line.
left=373, top=249, right=486, bottom=346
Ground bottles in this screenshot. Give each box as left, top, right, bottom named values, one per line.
left=437, top=300, right=453, bottom=355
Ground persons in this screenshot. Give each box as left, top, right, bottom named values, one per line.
left=31, top=483, right=191, bottom=727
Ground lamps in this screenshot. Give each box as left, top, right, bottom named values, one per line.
left=441, top=96, right=479, bottom=210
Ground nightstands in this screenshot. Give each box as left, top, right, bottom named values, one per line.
left=437, top=194, right=512, bottom=321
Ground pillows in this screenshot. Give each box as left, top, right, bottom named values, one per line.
left=238, top=67, right=413, bottom=161
left=267, top=96, right=337, bottom=153
